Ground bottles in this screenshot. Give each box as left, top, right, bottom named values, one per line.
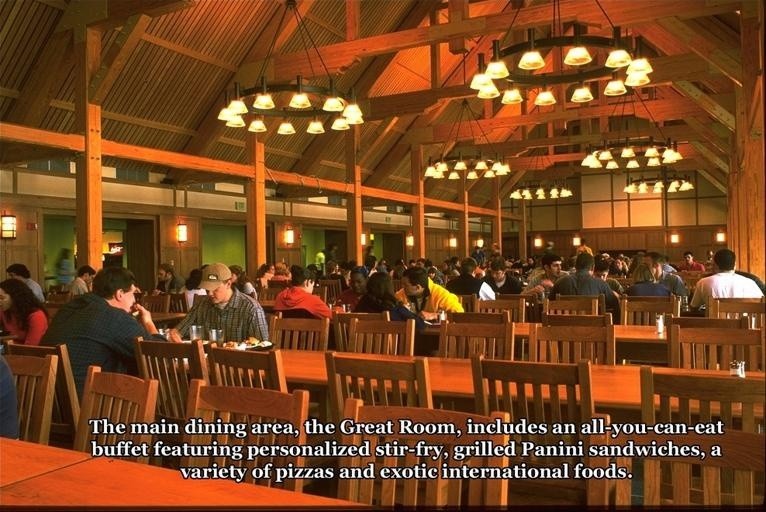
left=439, top=309, right=447, bottom=325
left=655, top=315, right=665, bottom=334
left=729, top=360, right=746, bottom=378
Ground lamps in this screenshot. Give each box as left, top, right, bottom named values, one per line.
left=406, top=231, right=414, bottom=250
left=217, top=0, right=365, bottom=135
left=285, top=225, right=294, bottom=246
left=177, top=220, right=187, bottom=243
left=0, top=210, right=17, bottom=239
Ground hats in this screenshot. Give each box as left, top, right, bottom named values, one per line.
left=197, top=262, right=232, bottom=291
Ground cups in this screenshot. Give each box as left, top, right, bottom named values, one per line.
left=207, top=329, right=224, bottom=347
left=406, top=302, right=417, bottom=314
left=189, top=323, right=203, bottom=342
left=156, top=328, right=170, bottom=342
left=341, top=304, right=352, bottom=313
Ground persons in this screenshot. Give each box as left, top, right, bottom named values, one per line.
left=0, top=353, right=22, bottom=441
left=37, top=266, right=168, bottom=419
left=1, top=240, right=766, bottom=349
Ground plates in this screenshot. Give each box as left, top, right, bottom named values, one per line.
left=244, top=343, right=275, bottom=352
